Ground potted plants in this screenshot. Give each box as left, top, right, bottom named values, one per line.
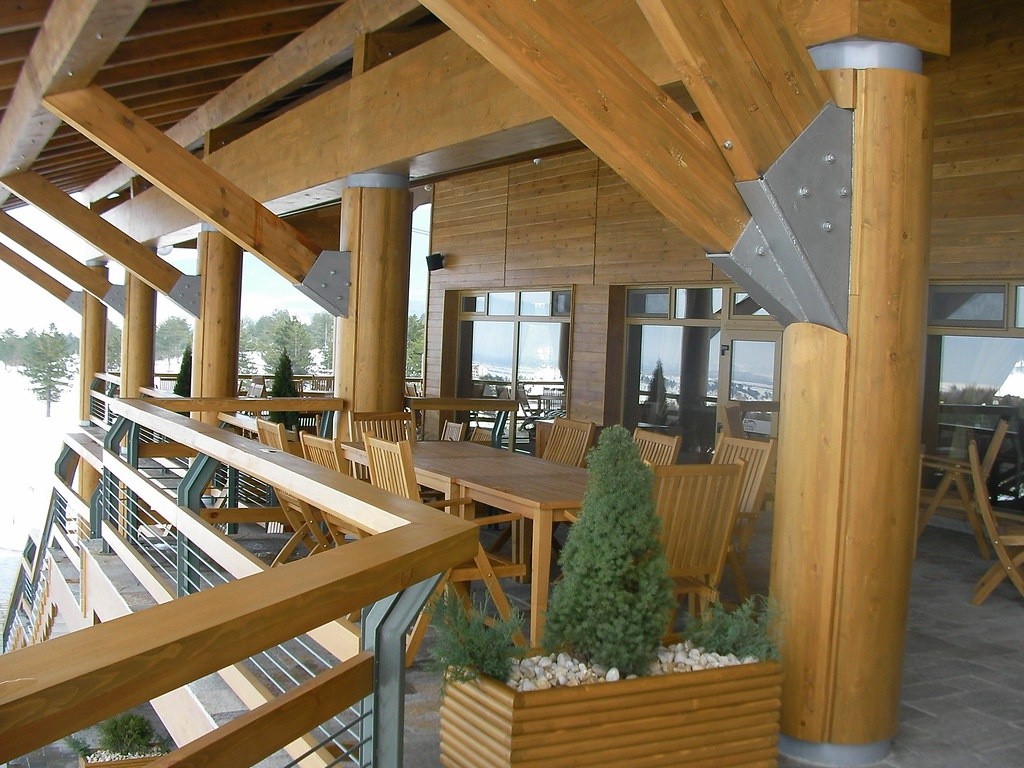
left=423, top=422, right=785, bottom=768
left=64, top=714, right=174, bottom=768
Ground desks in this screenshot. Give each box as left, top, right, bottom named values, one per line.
left=340, top=439, right=589, bottom=650
left=298, top=392, right=334, bottom=398
left=526, top=394, right=566, bottom=415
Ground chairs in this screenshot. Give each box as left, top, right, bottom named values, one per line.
left=235, top=373, right=1023, bottom=673
left=466, top=383, right=486, bottom=427
left=495, top=386, right=510, bottom=399
left=514, top=385, right=544, bottom=430
